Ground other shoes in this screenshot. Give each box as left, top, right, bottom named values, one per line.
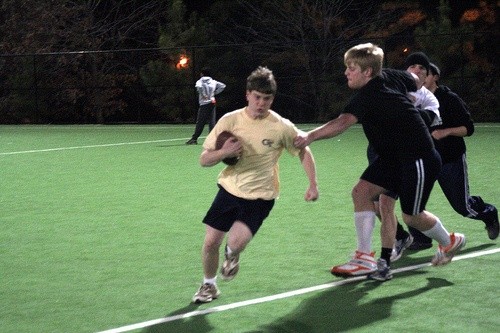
left=187, top=138, right=197, bottom=145
left=484, top=206, right=499, bottom=239
left=406, top=239, right=432, bottom=251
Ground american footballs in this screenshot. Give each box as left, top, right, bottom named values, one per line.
left=216, top=130, right=239, bottom=166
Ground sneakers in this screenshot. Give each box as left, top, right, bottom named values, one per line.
left=220, top=243, right=240, bottom=281
left=368, top=258, right=393, bottom=281
left=391, top=230, right=414, bottom=260
left=330, top=249, right=377, bottom=276
left=192, top=282, right=220, bottom=303
left=431, top=232, right=466, bottom=266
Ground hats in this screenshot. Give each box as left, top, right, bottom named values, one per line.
left=404, top=51, right=430, bottom=77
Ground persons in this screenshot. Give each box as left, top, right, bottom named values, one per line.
left=292, top=42, right=467, bottom=276
left=189, top=66, right=320, bottom=303
left=362, top=50, right=443, bottom=281
left=186, top=71, right=226, bottom=146
left=405, top=63, right=499, bottom=252
